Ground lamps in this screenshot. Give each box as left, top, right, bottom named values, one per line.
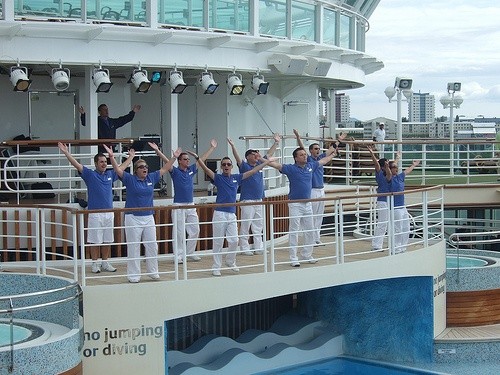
left=167, top=67, right=188, bottom=95
left=126, top=66, right=153, bottom=95
left=8, top=62, right=33, bottom=92
left=199, top=69, right=219, bottom=95
left=92, top=64, right=114, bottom=93
left=225, top=70, right=246, bottom=95
left=250, top=71, right=270, bottom=96
left=51, top=64, right=71, bottom=92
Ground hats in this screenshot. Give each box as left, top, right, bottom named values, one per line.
left=379, top=122, right=384, bottom=126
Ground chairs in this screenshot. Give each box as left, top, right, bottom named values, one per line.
left=43, top=2, right=146, bottom=22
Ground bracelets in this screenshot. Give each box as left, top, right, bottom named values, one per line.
left=338, top=140, right=342, bottom=144
left=195, top=155, right=199, bottom=160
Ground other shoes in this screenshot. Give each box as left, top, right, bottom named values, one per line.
left=240, top=250, right=253, bottom=256
left=314, top=240, right=326, bottom=248
left=290, top=262, right=300, bottom=267
left=178, top=256, right=183, bottom=264
left=91, top=262, right=101, bottom=273
left=226, top=263, right=239, bottom=272
left=254, top=250, right=269, bottom=255
left=212, top=270, right=221, bottom=276
left=372, top=247, right=383, bottom=252
left=129, top=278, right=139, bottom=283
left=148, top=274, right=160, bottom=279
left=305, top=257, right=319, bottom=263
left=187, top=256, right=202, bottom=260
left=101, top=263, right=117, bottom=272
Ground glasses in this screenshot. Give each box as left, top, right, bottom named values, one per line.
left=183, top=158, right=190, bottom=160
left=314, top=148, right=320, bottom=150
left=137, top=165, right=148, bottom=169
left=221, top=163, right=232, bottom=166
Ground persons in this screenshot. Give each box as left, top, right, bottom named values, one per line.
left=103, top=144, right=182, bottom=282
left=79, top=103, right=141, bottom=200
left=294, top=129, right=347, bottom=247
left=367, top=145, right=401, bottom=252
left=227, top=132, right=280, bottom=255
left=148, top=139, right=217, bottom=263
left=253, top=147, right=338, bottom=267
left=372, top=122, right=385, bottom=159
left=454, top=161, right=467, bottom=175
left=385, top=160, right=420, bottom=255
left=186, top=151, right=278, bottom=275
left=58, top=141, right=135, bottom=272
